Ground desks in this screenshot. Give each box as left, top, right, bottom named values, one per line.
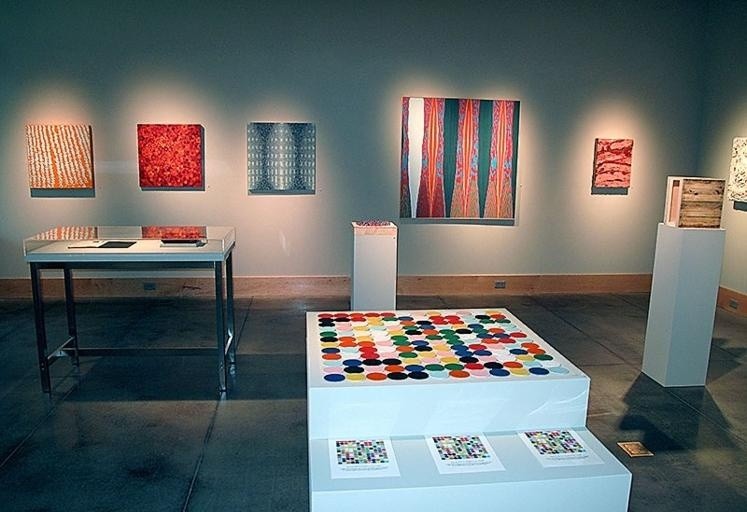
left=24, top=225, right=235, bottom=392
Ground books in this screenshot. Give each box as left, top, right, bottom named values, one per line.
left=159, top=239, right=201, bottom=248
